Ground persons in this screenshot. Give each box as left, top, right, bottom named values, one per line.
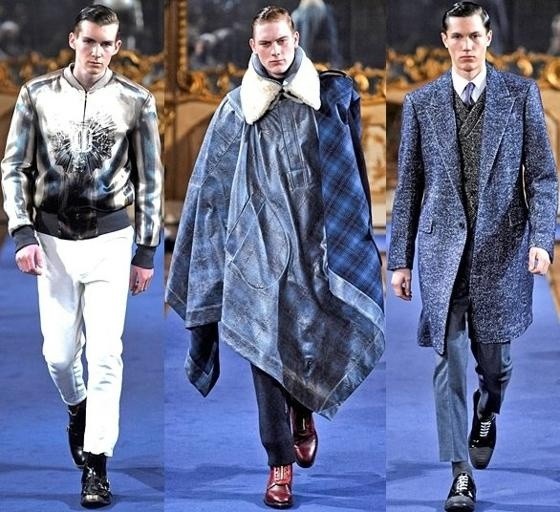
left=387, top=1, right=558, bottom=511
left=163, top=6, right=385, bottom=508
left=0, top=5, right=166, bottom=509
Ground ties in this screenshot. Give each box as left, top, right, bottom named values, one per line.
left=463, top=83, right=475, bottom=110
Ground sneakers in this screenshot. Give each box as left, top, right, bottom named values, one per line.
left=265, top=463, right=294, bottom=509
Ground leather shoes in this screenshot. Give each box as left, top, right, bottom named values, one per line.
left=80, top=452, right=113, bottom=506
left=289, top=402, right=319, bottom=468
left=468, top=390, right=497, bottom=469
left=67, top=398, right=90, bottom=468
left=444, top=473, right=476, bottom=510
